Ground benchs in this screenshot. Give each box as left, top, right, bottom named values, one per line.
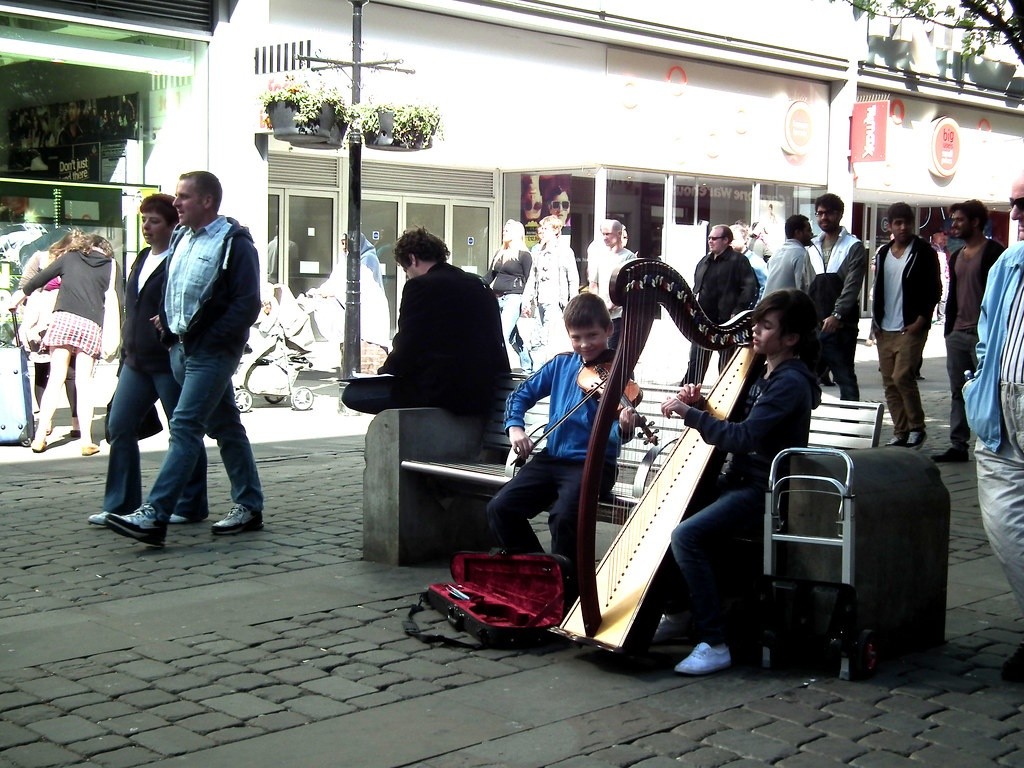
left=401, top=374, right=884, bottom=504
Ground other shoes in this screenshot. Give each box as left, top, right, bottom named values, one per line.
left=1000, top=641, right=1024, bottom=680
left=169, top=514, right=186, bottom=522
left=885, top=436, right=906, bottom=447
left=907, top=432, right=927, bottom=450
left=87, top=511, right=109, bottom=525
left=82, top=444, right=100, bottom=455
left=931, top=447, right=968, bottom=461
left=31, top=441, right=47, bottom=453
left=70, top=430, right=80, bottom=438
left=821, top=373, right=836, bottom=387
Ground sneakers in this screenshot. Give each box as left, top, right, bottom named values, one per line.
left=675, top=641, right=731, bottom=674
left=212, top=503, right=263, bottom=535
left=106, top=504, right=170, bottom=548
left=651, top=617, right=692, bottom=652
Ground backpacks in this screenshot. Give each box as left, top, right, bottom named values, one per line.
left=810, top=272, right=844, bottom=329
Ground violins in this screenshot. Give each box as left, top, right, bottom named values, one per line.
left=577, top=361, right=662, bottom=447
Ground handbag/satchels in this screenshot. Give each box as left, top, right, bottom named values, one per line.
left=100, top=288, right=121, bottom=358
left=105, top=397, right=163, bottom=445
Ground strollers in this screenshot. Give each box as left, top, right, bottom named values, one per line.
left=234, top=281, right=316, bottom=413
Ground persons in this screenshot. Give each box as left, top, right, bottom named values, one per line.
left=485, top=293, right=636, bottom=646
left=267, top=221, right=301, bottom=296
left=339, top=225, right=507, bottom=419
left=766, top=204, right=776, bottom=225
left=523, top=174, right=571, bottom=226
left=481, top=190, right=1006, bottom=464
left=649, top=288, right=823, bottom=675
left=5, top=171, right=389, bottom=547
left=962, top=175, right=1023, bottom=685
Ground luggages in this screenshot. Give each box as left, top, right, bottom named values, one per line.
left=0, top=306, right=35, bottom=447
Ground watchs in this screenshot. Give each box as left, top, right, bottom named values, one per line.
left=831, top=311, right=843, bottom=321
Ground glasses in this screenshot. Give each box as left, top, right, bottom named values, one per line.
left=524, top=202, right=542, bottom=210
left=1009, top=198, right=1024, bottom=208
left=551, top=200, right=569, bottom=210
left=815, top=211, right=834, bottom=215
left=708, top=237, right=720, bottom=240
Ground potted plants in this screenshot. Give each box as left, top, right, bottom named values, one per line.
left=290, top=102, right=360, bottom=150
left=360, top=95, right=445, bottom=152
left=257, top=74, right=336, bottom=143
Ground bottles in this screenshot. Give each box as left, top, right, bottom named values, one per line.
left=961, top=370, right=977, bottom=394
